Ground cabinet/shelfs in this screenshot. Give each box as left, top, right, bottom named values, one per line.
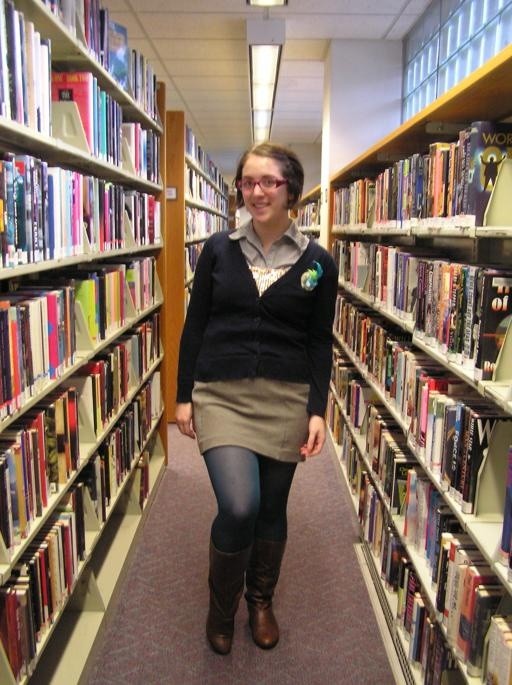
left=290, top=185, right=325, bottom=243
left=326, top=43, right=511, bottom=685
left=166, top=111, right=232, bottom=424
left=0, top=0, right=165, bottom=684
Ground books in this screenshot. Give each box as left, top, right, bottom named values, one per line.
left=185, top=124, right=231, bottom=316
left=295, top=121, right=512, bottom=228
left=311, top=233, right=511, bottom=382
left=0, top=314, right=166, bottom=684
left=1, top=0, right=163, bottom=183
left=324, top=289, right=512, bottom=684
left=1, top=151, right=162, bottom=420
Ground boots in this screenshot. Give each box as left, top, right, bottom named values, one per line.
left=244, top=537, right=284, bottom=649
left=207, top=536, right=253, bottom=654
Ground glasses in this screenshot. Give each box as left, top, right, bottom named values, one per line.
left=235, top=178, right=287, bottom=189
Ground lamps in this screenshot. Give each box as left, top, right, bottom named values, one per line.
left=242, top=0, right=290, bottom=8
left=248, top=22, right=285, bottom=145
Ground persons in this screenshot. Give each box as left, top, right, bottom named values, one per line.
left=171, top=141, right=344, bottom=656
